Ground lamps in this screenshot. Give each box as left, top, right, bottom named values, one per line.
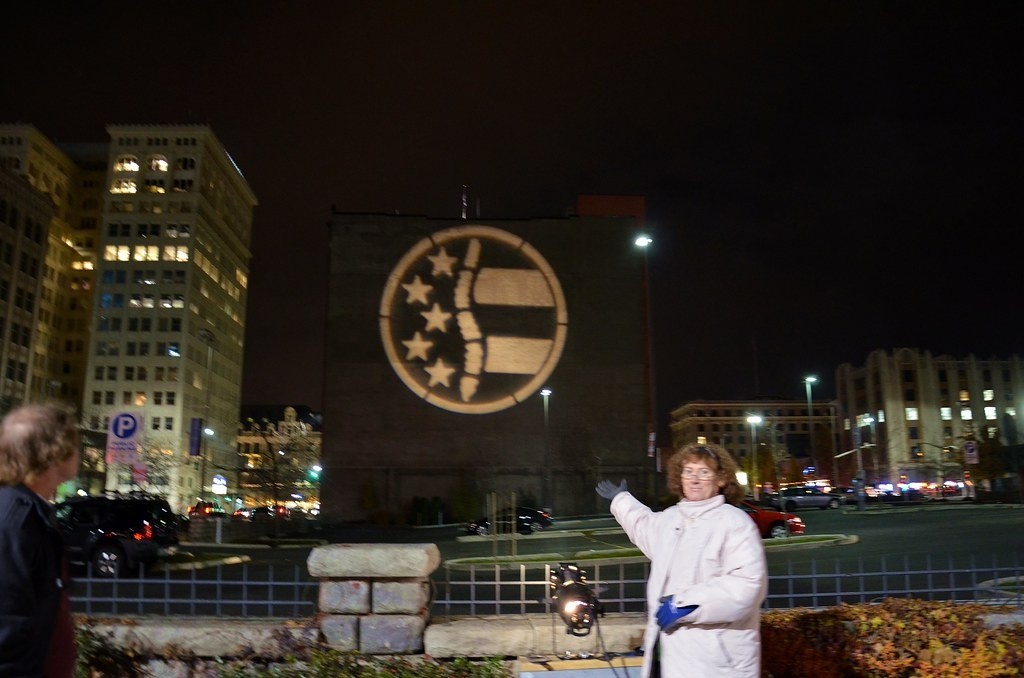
left=547, top=559, right=601, bottom=635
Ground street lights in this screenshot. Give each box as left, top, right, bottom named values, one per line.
left=746, top=415, right=763, bottom=499
left=634, top=232, right=657, bottom=508
left=805, top=374, right=819, bottom=477
left=538, top=387, right=557, bottom=515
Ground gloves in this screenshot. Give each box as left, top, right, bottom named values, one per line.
left=655, top=595, right=698, bottom=630
left=595, top=479, right=628, bottom=500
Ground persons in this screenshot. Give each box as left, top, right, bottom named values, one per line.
left=0, top=401, right=82, bottom=678
left=595, top=442, right=768, bottom=678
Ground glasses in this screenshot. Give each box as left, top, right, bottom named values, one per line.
left=679, top=469, right=717, bottom=481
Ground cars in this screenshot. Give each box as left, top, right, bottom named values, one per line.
left=770, top=484, right=847, bottom=512
left=50, top=489, right=295, bottom=578
left=465, top=506, right=554, bottom=536
left=828, top=485, right=858, bottom=504
left=737, top=499, right=806, bottom=539
